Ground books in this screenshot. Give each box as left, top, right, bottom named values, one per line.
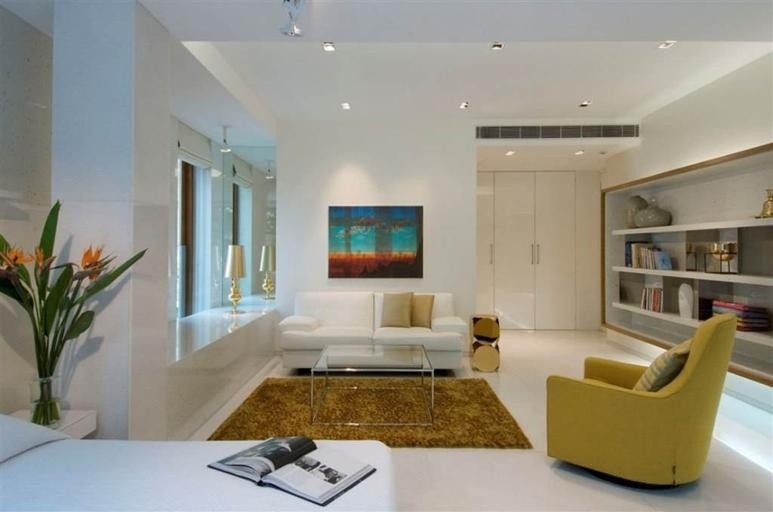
left=711, top=298, right=769, bottom=333
left=623, top=240, right=673, bottom=271
left=207, top=437, right=379, bottom=505
left=638, top=287, right=663, bottom=313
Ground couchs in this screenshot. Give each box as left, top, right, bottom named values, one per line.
left=278, top=291, right=469, bottom=374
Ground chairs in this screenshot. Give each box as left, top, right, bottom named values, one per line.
left=545, top=312, right=737, bottom=487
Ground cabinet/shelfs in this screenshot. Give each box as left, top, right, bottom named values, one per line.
left=599, top=142, right=773, bottom=387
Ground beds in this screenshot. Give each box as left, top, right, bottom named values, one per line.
left=0, top=437, right=396, bottom=510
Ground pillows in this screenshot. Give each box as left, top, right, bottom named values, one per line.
left=630, top=338, right=693, bottom=392
left=0, top=414, right=67, bottom=463
left=379, top=292, right=434, bottom=329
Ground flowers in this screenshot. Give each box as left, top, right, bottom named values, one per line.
left=0, top=200, right=148, bottom=426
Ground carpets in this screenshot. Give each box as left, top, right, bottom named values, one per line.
left=204, top=376, right=534, bottom=449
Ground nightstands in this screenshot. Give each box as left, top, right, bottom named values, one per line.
left=9, top=409, right=98, bottom=439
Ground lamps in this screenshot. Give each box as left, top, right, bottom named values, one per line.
left=259, top=245, right=275, bottom=299
left=219, top=128, right=231, bottom=154
left=223, top=243, right=244, bottom=314
left=265, top=160, right=273, bottom=180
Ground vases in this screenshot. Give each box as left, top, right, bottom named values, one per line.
left=27, top=375, right=61, bottom=429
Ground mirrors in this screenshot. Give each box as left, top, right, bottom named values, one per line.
left=251, top=160, right=277, bottom=303
